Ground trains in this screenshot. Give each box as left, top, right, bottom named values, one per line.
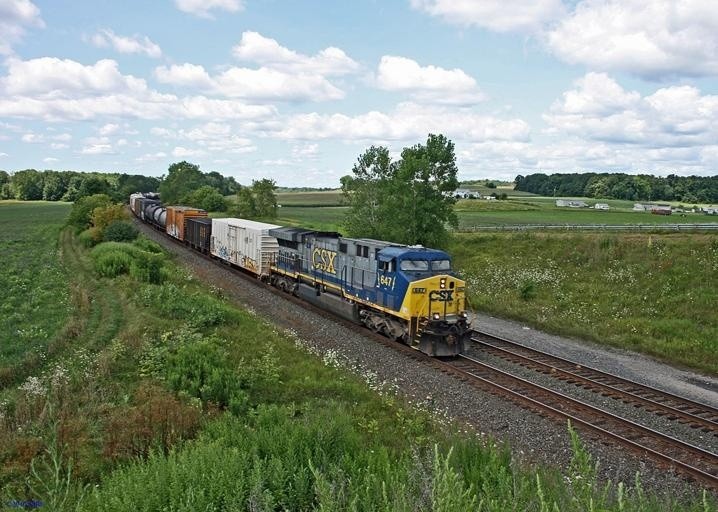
left=129, top=186, right=474, bottom=362
